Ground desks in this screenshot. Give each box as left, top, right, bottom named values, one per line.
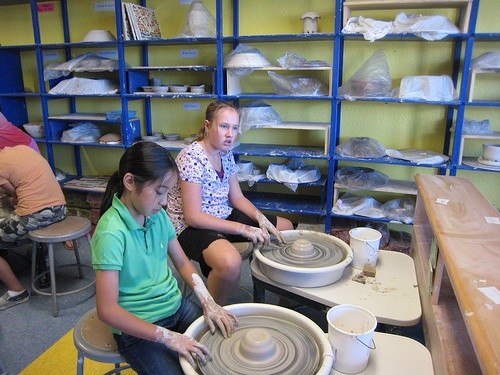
left=324, top=329, right=435, bottom=375
left=410, top=173, right=500, bottom=375
left=249, top=234, right=422, bottom=326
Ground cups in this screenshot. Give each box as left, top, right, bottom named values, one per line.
left=154, top=133, right=162, bottom=140
left=153, top=78, right=162, bottom=85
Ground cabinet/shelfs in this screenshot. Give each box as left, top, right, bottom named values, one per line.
left=0, top=0, right=500, bottom=236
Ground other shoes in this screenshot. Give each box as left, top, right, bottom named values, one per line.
left=64, top=238, right=80, bottom=250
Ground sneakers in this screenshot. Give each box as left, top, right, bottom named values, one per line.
left=0, top=289, right=31, bottom=311
left=38, top=267, right=49, bottom=288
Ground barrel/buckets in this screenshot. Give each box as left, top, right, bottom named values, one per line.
left=327, top=304, right=377, bottom=374
left=349, top=227, right=382, bottom=270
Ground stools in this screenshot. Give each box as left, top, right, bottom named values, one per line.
left=30, top=215, right=96, bottom=318
left=189, top=238, right=254, bottom=304
left=72, top=306, right=132, bottom=375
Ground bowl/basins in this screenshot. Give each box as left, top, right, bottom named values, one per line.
left=82, top=29, right=116, bottom=42
left=253, top=229, right=353, bottom=288
left=153, top=85, right=168, bottom=93
left=170, top=84, right=188, bottom=92
left=190, top=85, right=205, bottom=93
left=178, top=302, right=333, bottom=375
left=224, top=53, right=271, bottom=67
left=23, top=123, right=43, bottom=137
left=142, top=86, right=153, bottom=92
left=483, top=144, right=500, bottom=162
left=166, top=134, right=179, bottom=141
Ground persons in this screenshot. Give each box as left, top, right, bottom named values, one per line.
left=0, top=145, right=68, bottom=311
left=166, top=101, right=293, bottom=306
left=0, top=107, right=40, bottom=153
left=90, top=142, right=239, bottom=375
